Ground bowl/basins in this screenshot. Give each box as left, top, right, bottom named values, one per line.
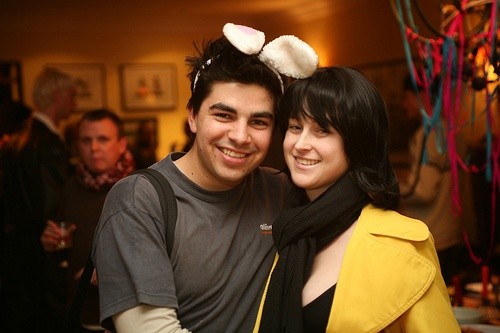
left=452, top=306, right=483, bottom=325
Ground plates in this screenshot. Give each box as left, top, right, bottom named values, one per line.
left=460, top=324, right=500, bottom=333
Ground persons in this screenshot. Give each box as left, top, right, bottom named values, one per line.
left=41, top=108, right=156, bottom=333
left=0, top=101, right=67, bottom=239
left=252, top=66, right=461, bottom=333
left=26, top=68, right=81, bottom=155
left=392, top=70, right=463, bottom=284
left=91, top=22, right=319, bottom=333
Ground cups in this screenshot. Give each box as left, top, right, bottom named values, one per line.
left=57, top=221, right=71, bottom=249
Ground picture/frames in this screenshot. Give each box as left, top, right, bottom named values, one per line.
left=118, top=62, right=180, bottom=113
left=43, top=62, right=108, bottom=113
